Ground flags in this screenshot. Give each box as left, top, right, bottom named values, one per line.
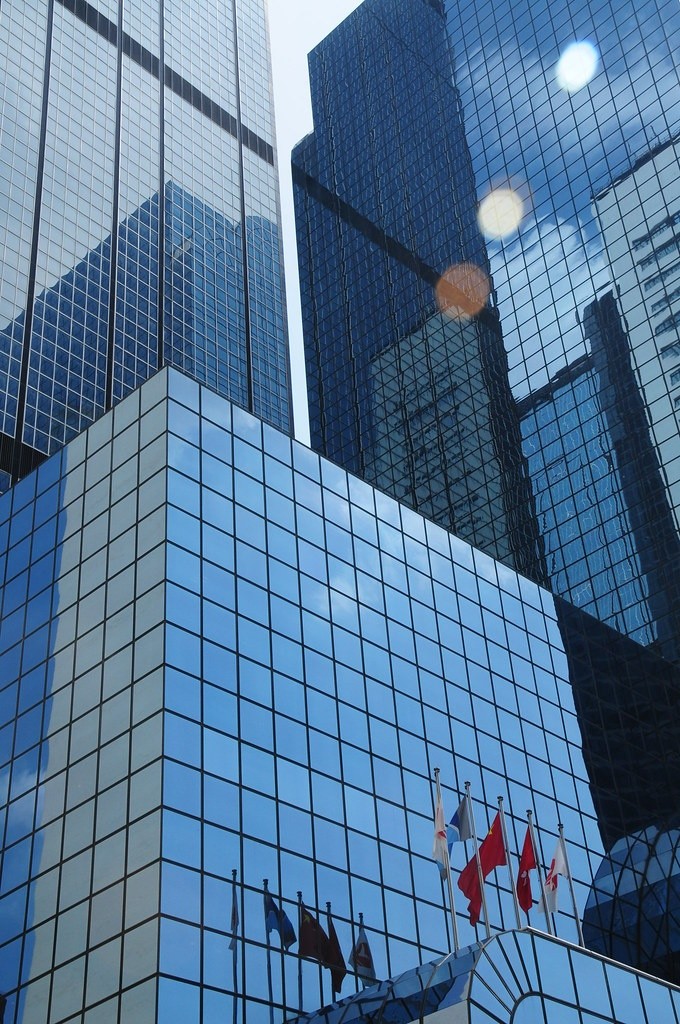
left=300, top=902, right=346, bottom=993
left=227, top=882, right=240, bottom=950
left=456, top=801, right=507, bottom=926
left=263, top=886, right=297, bottom=951
left=348, top=924, right=377, bottom=987
left=432, top=767, right=476, bottom=881
left=546, top=837, right=568, bottom=913
left=517, top=824, right=539, bottom=914
